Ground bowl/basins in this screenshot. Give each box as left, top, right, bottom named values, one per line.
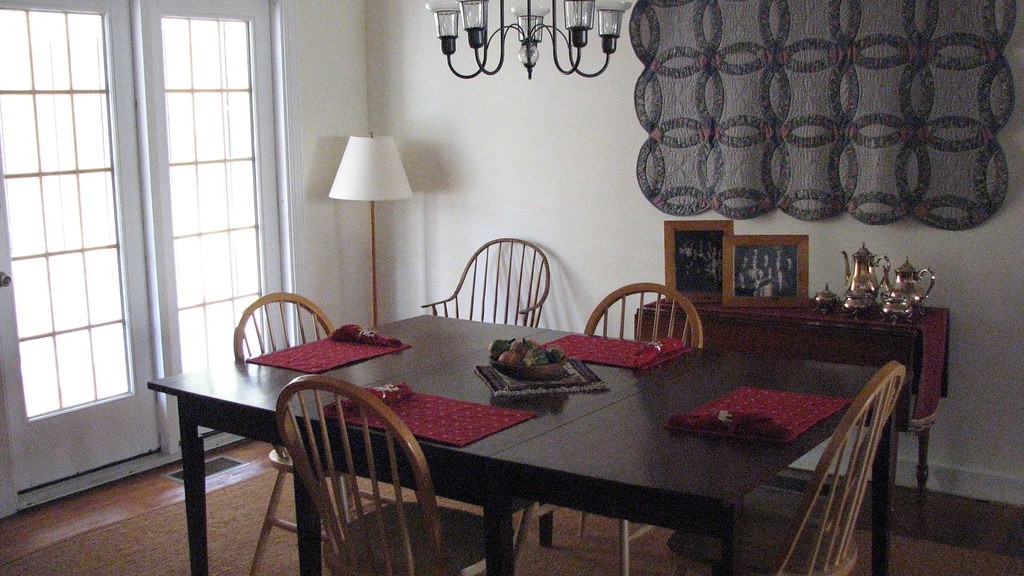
left=488, top=357, right=569, bottom=380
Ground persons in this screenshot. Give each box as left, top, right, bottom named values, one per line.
left=679, top=239, right=722, bottom=290
left=735, top=248, right=795, bottom=298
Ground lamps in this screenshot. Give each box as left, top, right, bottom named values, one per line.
left=328, top=131, right=412, bottom=328
left=425, top=0, right=632, bottom=80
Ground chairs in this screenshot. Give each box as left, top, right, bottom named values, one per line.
left=509, top=282, right=705, bottom=575
left=420, top=237, right=551, bottom=329
left=275, top=374, right=515, bottom=576
left=667, top=360, right=910, bottom=576
left=232, top=292, right=399, bottom=576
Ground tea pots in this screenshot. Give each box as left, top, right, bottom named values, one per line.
left=840, top=240, right=890, bottom=299
left=880, top=254, right=935, bottom=317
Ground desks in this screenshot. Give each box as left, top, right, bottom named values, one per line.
left=641, top=298, right=952, bottom=523
left=147, top=314, right=903, bottom=576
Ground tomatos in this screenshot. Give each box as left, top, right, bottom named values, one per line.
left=490, top=339, right=564, bottom=365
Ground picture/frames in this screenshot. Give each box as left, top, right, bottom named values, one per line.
left=722, top=233, right=810, bottom=310
left=664, top=219, right=735, bottom=303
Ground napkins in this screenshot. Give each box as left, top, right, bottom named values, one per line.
left=666, top=410, right=774, bottom=439
left=345, top=379, right=410, bottom=410
left=630, top=337, right=684, bottom=367
left=331, top=324, right=403, bottom=349
